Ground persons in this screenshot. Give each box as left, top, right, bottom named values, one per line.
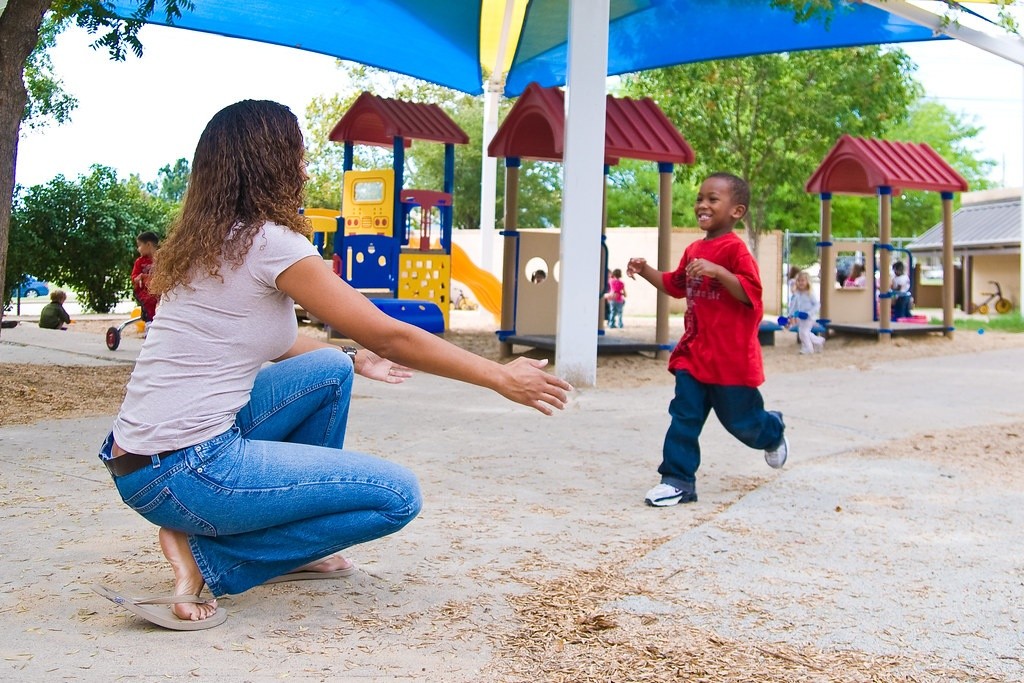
left=891, top=261, right=912, bottom=317
left=39, top=290, right=71, bottom=331
left=91, top=98, right=572, bottom=631
left=843, top=260, right=893, bottom=320
left=605, top=269, right=626, bottom=327
left=132, top=232, right=161, bottom=332
left=625, top=173, right=787, bottom=507
left=793, top=274, right=825, bottom=354
left=787, top=265, right=801, bottom=325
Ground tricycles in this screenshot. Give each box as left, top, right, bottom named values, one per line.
left=972, top=281, right=1012, bottom=315
left=106, top=275, right=151, bottom=351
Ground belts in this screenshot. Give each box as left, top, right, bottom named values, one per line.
left=98, top=446, right=187, bottom=477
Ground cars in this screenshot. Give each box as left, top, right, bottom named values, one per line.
left=11, top=275, right=49, bottom=299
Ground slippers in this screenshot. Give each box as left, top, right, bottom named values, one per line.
left=91, top=583, right=228, bottom=629
left=259, top=552, right=354, bottom=585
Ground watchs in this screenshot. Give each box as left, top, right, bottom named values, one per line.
left=341, top=345, right=357, bottom=363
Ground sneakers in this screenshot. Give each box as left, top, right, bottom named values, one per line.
left=645, top=483, right=686, bottom=506
left=764, top=437, right=788, bottom=467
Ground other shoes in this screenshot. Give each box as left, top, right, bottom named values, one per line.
left=799, top=349, right=814, bottom=355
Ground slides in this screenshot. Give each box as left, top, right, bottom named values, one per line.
left=434, top=238, right=502, bottom=323
